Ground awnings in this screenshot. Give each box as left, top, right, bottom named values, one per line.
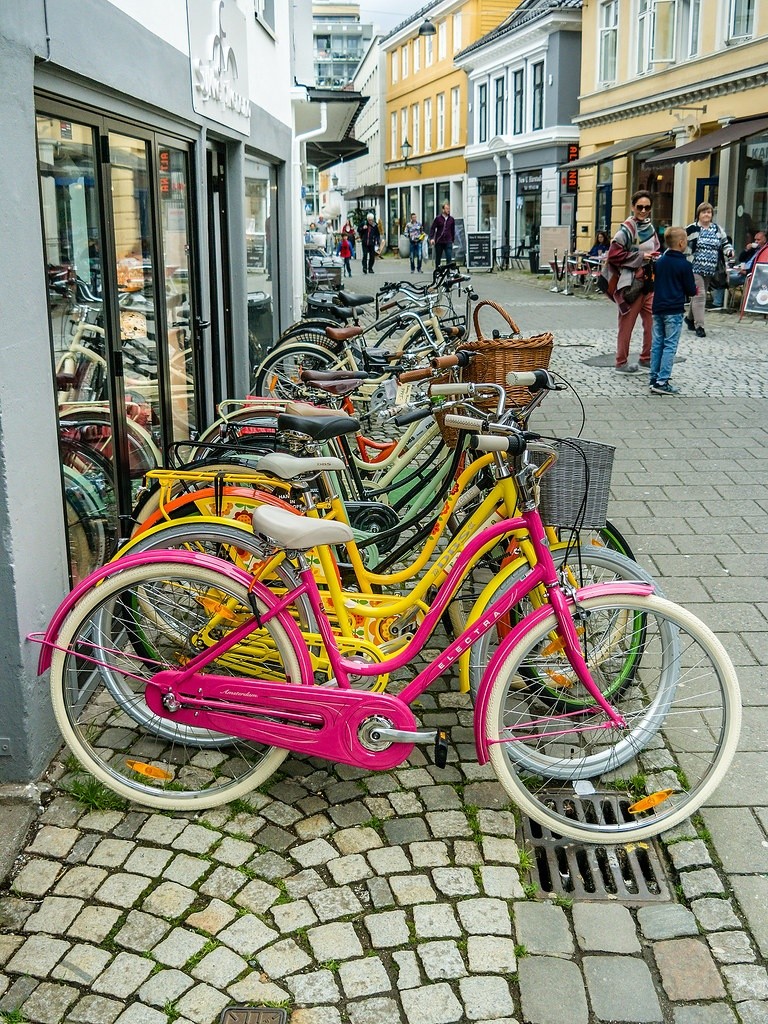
left=643, top=112, right=768, bottom=171
left=556, top=130, right=676, bottom=172
left=306, top=136, right=368, bottom=172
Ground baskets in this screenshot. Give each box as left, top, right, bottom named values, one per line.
left=428, top=375, right=526, bottom=446
left=507, top=436, right=616, bottom=530
left=455, top=300, right=554, bottom=407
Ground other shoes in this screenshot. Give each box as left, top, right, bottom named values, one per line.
left=638, top=359, right=651, bottom=369
left=411, top=269, right=415, bottom=274
left=418, top=268, right=424, bottom=274
left=684, top=317, right=695, bottom=331
left=348, top=271, right=352, bottom=278
left=363, top=267, right=367, bottom=274
left=368, top=267, right=374, bottom=273
left=696, top=327, right=706, bottom=337
left=615, top=362, right=638, bottom=373
left=704, top=302, right=722, bottom=312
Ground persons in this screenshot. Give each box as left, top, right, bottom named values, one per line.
left=606, top=190, right=660, bottom=371
left=723, top=235, right=732, bottom=262
left=403, top=213, right=423, bottom=274
left=429, top=205, right=455, bottom=279
left=574, top=232, right=611, bottom=288
left=266, top=216, right=272, bottom=281
left=306, top=215, right=356, bottom=278
left=654, top=220, right=669, bottom=255
left=705, top=232, right=768, bottom=311
left=76, top=239, right=103, bottom=303
left=684, top=202, right=735, bottom=336
left=649, top=227, right=697, bottom=395
left=357, top=213, right=380, bottom=273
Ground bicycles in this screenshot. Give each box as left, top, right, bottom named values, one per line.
left=28, top=260, right=743, bottom=845
left=45, top=254, right=196, bottom=601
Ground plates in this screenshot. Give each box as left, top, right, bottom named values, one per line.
left=573, top=252, right=583, bottom=255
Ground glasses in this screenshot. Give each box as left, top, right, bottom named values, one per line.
left=634, top=204, right=653, bottom=212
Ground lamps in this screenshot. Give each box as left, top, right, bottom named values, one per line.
left=399, top=138, right=422, bottom=173
left=418, top=17, right=437, bottom=36
left=332, top=173, right=345, bottom=195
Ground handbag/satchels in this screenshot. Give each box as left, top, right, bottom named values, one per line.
left=358, top=221, right=367, bottom=240
left=709, top=242, right=730, bottom=291
left=422, top=234, right=428, bottom=264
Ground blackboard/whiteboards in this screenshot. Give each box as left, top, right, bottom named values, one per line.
left=539, top=225, right=571, bottom=269
left=433, top=218, right=465, bottom=260
left=465, top=231, right=493, bottom=268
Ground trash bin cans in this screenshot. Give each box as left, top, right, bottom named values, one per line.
left=305, top=291, right=344, bottom=324
left=529, top=250, right=550, bottom=274
left=247, top=290, right=273, bottom=391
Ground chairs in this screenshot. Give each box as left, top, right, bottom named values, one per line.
left=549, top=253, right=609, bottom=298
left=492, top=245, right=524, bottom=271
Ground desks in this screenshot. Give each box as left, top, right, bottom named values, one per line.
left=311, top=264, right=342, bottom=298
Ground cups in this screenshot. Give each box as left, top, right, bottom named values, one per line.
left=598, top=250, right=602, bottom=257
left=751, top=243, right=758, bottom=248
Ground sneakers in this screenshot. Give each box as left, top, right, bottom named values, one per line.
left=652, top=380, right=678, bottom=395
left=649, top=378, right=657, bottom=389
